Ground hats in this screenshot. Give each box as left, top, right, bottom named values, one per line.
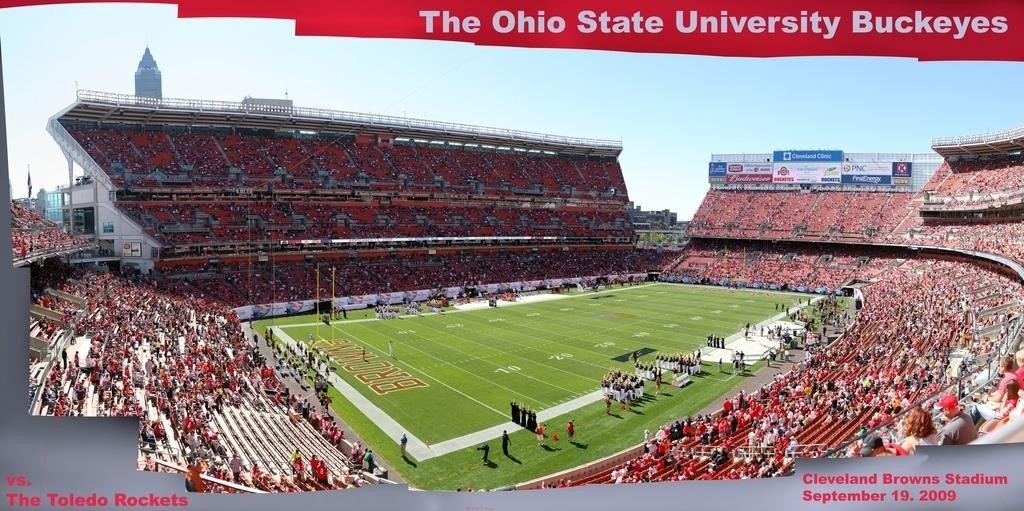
left=857, top=434, right=884, bottom=459
left=931, top=394, right=959, bottom=412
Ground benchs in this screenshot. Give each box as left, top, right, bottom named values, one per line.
left=8, top=120, right=1023, bottom=297
left=29, top=296, right=367, bottom=491
left=521, top=297, right=1022, bottom=490
